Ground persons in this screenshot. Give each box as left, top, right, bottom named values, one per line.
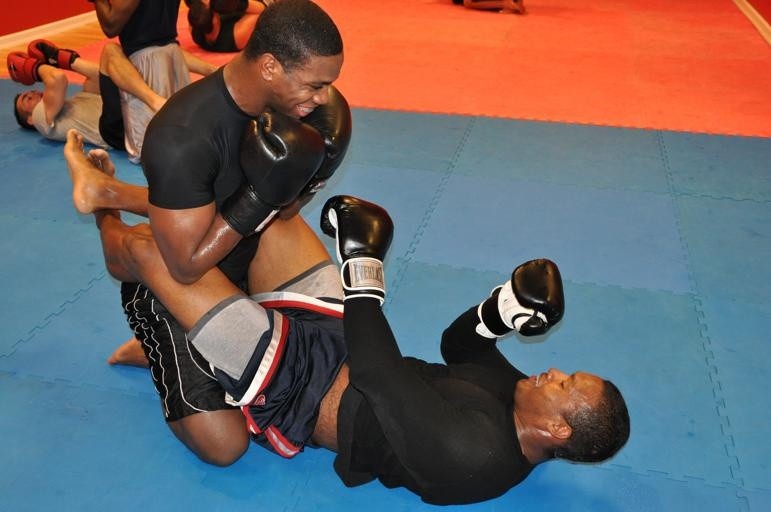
left=450, top=0, right=526, bottom=16
left=90, top=1, right=193, bottom=163
left=186, top=1, right=279, bottom=51
left=6, top=39, right=220, bottom=151
left=104, top=1, right=345, bottom=466
left=62, top=129, right=630, bottom=506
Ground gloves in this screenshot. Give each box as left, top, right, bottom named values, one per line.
left=7, top=39, right=79, bottom=86
left=478, top=259, right=564, bottom=336
left=221, top=84, right=352, bottom=236
left=321, top=195, right=394, bottom=304
